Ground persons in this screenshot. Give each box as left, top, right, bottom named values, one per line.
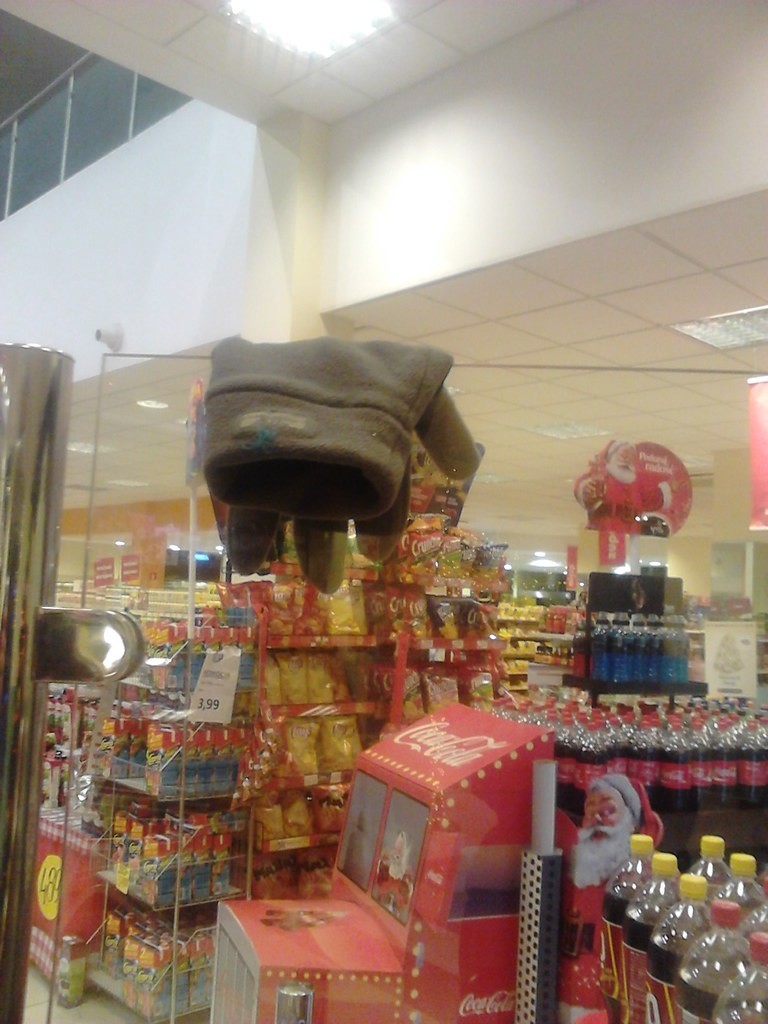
left=374, top=830, right=416, bottom=920
left=554, top=773, right=666, bottom=1023
left=573, top=440, right=679, bottom=535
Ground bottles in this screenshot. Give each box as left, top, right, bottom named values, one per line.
left=535, top=604, right=689, bottom=683
left=597, top=834, right=768, bottom=1024
left=468, top=693, right=767, bottom=816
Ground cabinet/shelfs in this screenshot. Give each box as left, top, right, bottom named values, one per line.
left=492, top=611, right=768, bottom=697
left=82, top=616, right=506, bottom=1023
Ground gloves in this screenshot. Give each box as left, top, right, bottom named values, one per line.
left=203, top=336, right=486, bottom=596
